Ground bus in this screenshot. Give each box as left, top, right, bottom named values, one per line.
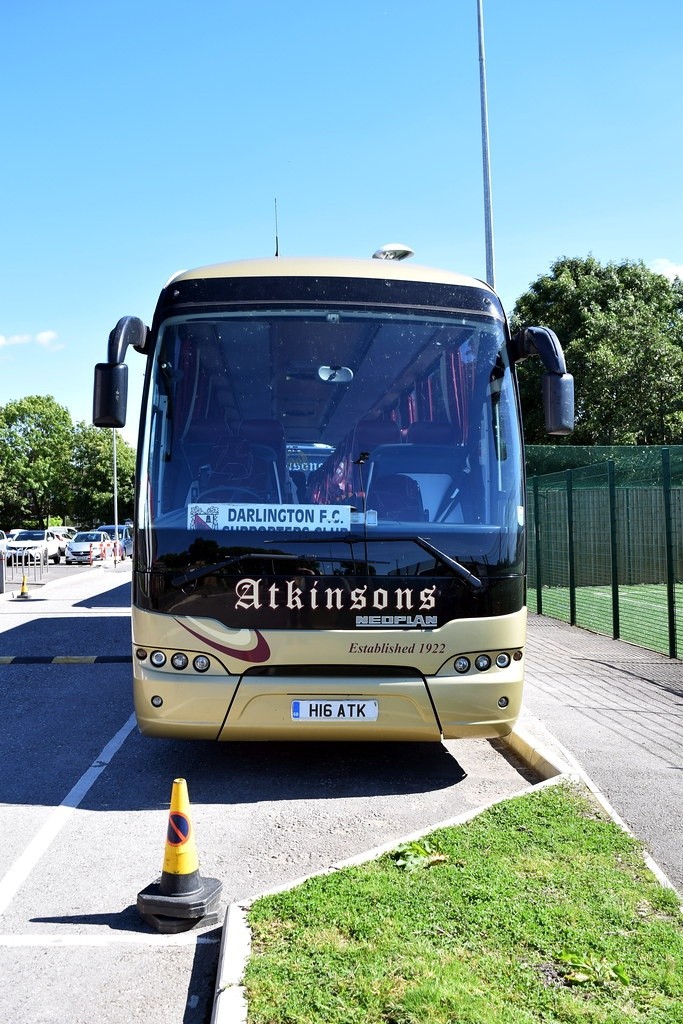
left=95, top=257, right=575, bottom=751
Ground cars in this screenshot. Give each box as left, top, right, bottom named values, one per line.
left=5, top=530, right=63, bottom=565
left=64, top=531, right=111, bottom=564
left=46, top=526, right=80, bottom=539
left=0, top=528, right=9, bottom=558
left=96, top=525, right=135, bottom=560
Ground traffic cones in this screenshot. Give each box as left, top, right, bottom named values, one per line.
left=16, top=575, right=32, bottom=599
left=130, top=774, right=225, bottom=923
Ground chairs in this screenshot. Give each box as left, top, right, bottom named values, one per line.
left=307, top=420, right=478, bottom=523
left=238, top=418, right=285, bottom=497
left=184, top=434, right=271, bottom=510
left=172, top=396, right=234, bottom=478
